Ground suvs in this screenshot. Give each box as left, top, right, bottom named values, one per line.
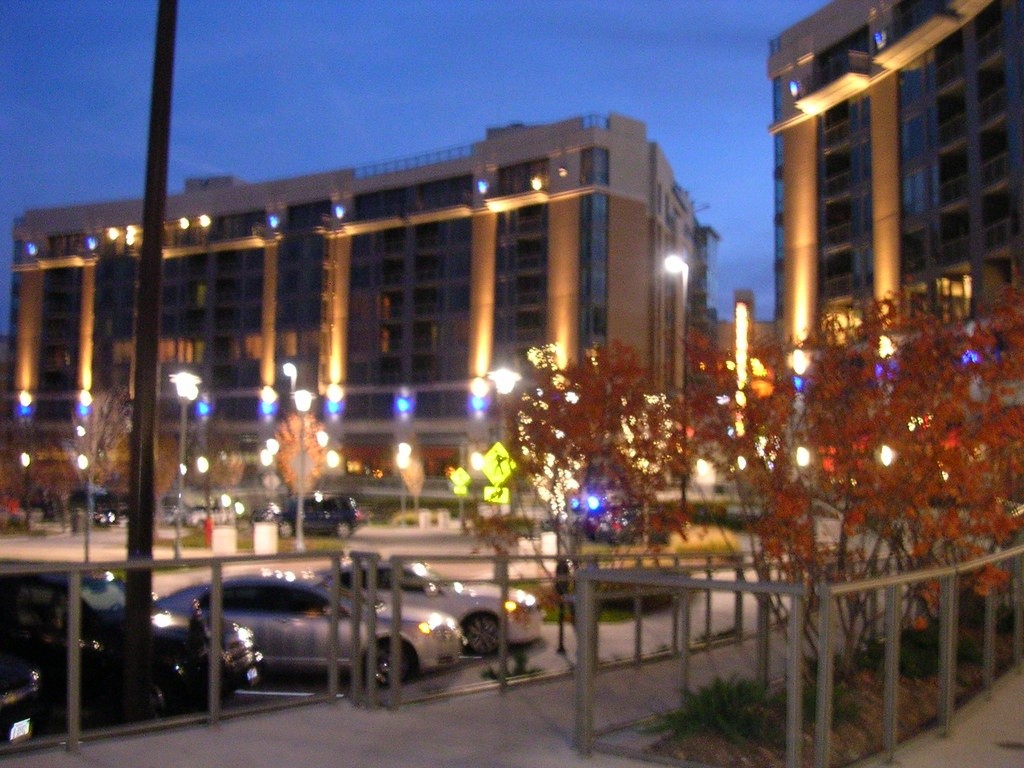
left=254, top=491, right=363, bottom=538
left=0, top=557, right=265, bottom=714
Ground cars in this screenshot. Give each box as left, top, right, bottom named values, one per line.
left=317, top=562, right=546, bottom=658
left=0, top=653, right=48, bottom=742
left=152, top=572, right=463, bottom=689
left=586, top=502, right=678, bottom=544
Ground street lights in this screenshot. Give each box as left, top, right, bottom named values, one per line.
left=665, top=249, right=687, bottom=513
left=169, top=368, right=200, bottom=559
left=20, top=390, right=35, bottom=531
left=292, top=390, right=312, bottom=547
left=489, top=361, right=522, bottom=586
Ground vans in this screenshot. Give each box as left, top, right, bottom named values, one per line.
left=43, top=484, right=116, bottom=527
